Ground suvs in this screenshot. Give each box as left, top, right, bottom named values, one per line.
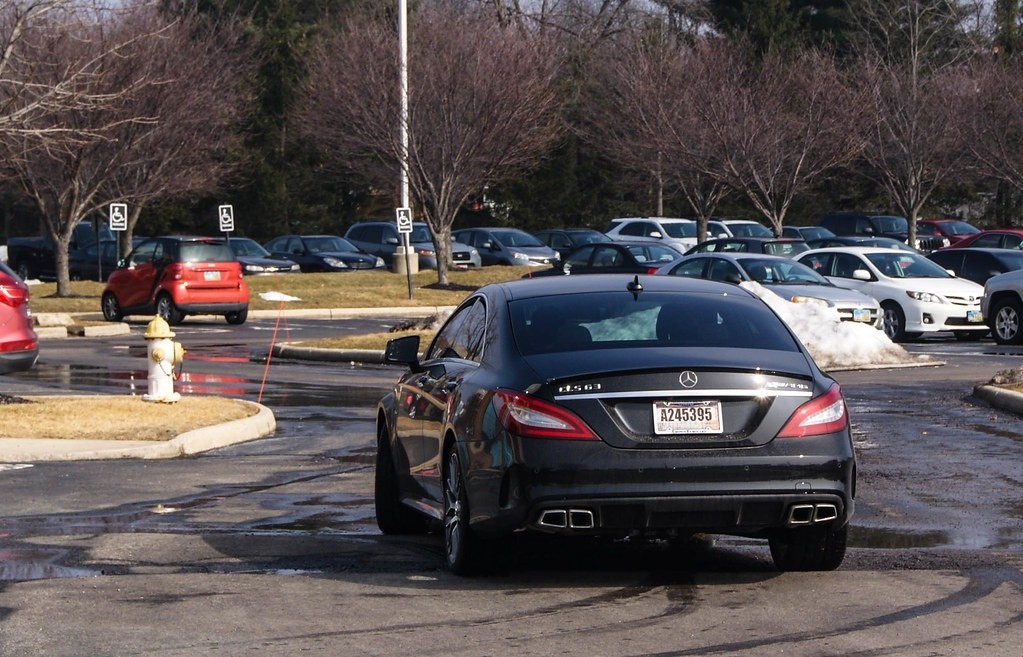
left=343, top=221, right=485, bottom=271
left=0, top=260, right=41, bottom=378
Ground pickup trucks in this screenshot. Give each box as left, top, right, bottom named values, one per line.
left=3, top=217, right=111, bottom=279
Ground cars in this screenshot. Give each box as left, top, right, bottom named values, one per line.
left=98, top=234, right=254, bottom=328
left=682, top=236, right=814, bottom=259
left=938, top=228, right=1023, bottom=252
left=371, top=269, right=858, bottom=571
left=650, top=251, right=886, bottom=334
left=977, top=271, right=1023, bottom=346
left=515, top=238, right=685, bottom=279
left=697, top=217, right=778, bottom=248
left=815, top=210, right=952, bottom=258
left=913, top=218, right=982, bottom=245
left=264, top=232, right=378, bottom=275
left=603, top=216, right=737, bottom=262
left=216, top=235, right=299, bottom=278
left=59, top=236, right=157, bottom=282
left=758, top=224, right=835, bottom=255
left=440, top=225, right=561, bottom=272
left=925, top=244, right=1023, bottom=287
left=528, top=224, right=615, bottom=255
left=791, top=245, right=993, bottom=342
